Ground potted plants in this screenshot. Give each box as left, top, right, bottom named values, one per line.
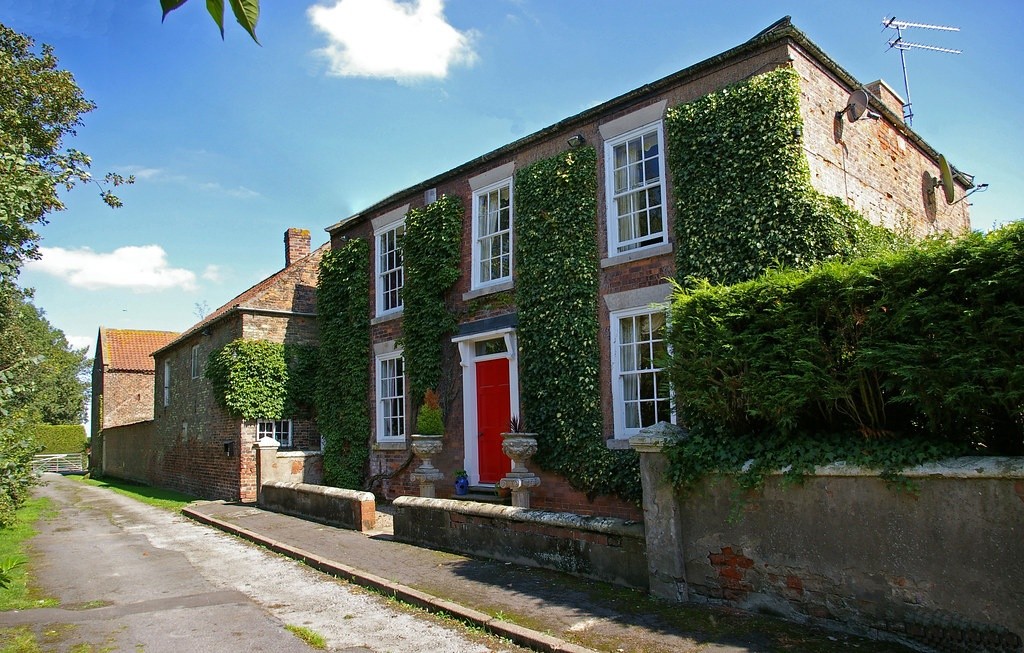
left=409, top=388, right=446, bottom=473
left=454, top=467, right=470, bottom=495
left=500, top=410, right=539, bottom=479
left=494, top=481, right=511, bottom=498
